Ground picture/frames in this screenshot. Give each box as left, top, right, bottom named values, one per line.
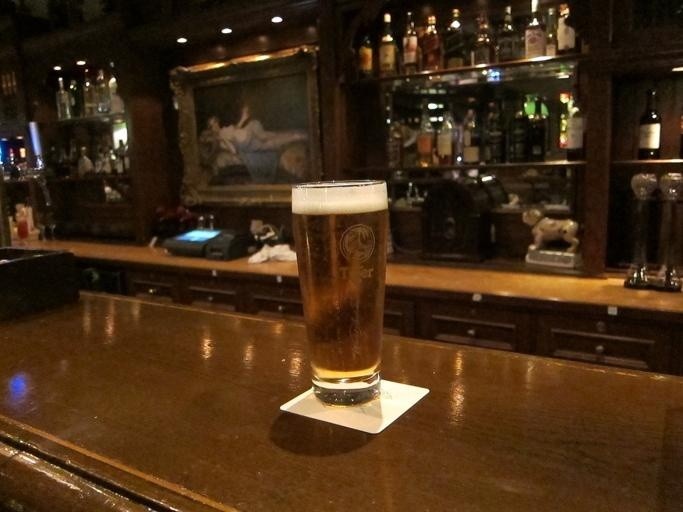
left=172, top=43, right=324, bottom=210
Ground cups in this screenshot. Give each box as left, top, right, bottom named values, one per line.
left=14, top=213, right=29, bottom=247
left=288, top=178, right=390, bottom=408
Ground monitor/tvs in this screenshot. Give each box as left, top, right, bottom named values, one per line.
left=164, top=228, right=223, bottom=255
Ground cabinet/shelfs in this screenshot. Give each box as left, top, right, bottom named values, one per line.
left=336, top=49, right=584, bottom=174
left=83, top=261, right=682, bottom=377
left=36, top=45, right=177, bottom=247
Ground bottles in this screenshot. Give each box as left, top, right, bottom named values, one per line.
left=356, top=0, right=577, bottom=79
left=53, top=64, right=124, bottom=121
left=387, top=78, right=588, bottom=169
left=639, top=87, right=662, bottom=161
left=59, top=134, right=131, bottom=177
